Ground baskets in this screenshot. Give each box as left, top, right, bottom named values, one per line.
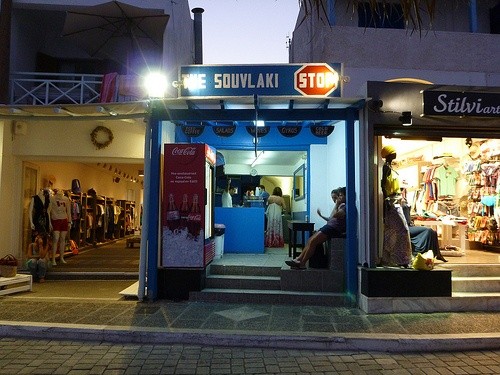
left=0, top=254, right=17, bottom=277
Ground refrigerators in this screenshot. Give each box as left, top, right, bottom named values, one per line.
left=162, top=143, right=216, bottom=267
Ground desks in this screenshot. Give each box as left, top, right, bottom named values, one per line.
left=286, top=220, right=315, bottom=259
left=126, top=238, right=140, bottom=248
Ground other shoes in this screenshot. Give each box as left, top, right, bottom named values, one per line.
left=38, top=277, right=45, bottom=283
left=285, top=259, right=306, bottom=270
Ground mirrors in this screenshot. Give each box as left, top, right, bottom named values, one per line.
left=294, top=164, right=305, bottom=201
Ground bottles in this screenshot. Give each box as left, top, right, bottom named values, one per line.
left=166, top=194, right=180, bottom=235
left=179, top=193, right=190, bottom=234
left=187, top=195, right=201, bottom=241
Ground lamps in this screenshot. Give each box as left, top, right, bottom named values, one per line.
left=399, top=111, right=414, bottom=127
left=97, top=163, right=144, bottom=185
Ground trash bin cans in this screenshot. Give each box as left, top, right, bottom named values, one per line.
left=214, top=224, right=226, bottom=259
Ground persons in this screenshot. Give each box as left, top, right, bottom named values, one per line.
left=401, top=198, right=448, bottom=263
left=30, top=187, right=71, bottom=265
left=381, top=145, right=414, bottom=267
left=265, top=187, right=286, bottom=248
left=285, top=187, right=346, bottom=269
left=222, top=175, right=236, bottom=207
left=258, top=185, right=269, bottom=209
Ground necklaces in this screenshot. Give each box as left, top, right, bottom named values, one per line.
left=385, top=163, right=399, bottom=175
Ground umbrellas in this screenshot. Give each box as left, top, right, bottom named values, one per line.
left=61, top=0, right=170, bottom=77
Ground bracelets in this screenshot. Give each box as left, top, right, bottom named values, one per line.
left=68, top=222, right=71, bottom=223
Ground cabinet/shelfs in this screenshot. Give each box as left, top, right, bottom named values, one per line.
left=52, top=190, right=136, bottom=249
left=0, top=274, right=32, bottom=295
left=413, top=219, right=467, bottom=257
